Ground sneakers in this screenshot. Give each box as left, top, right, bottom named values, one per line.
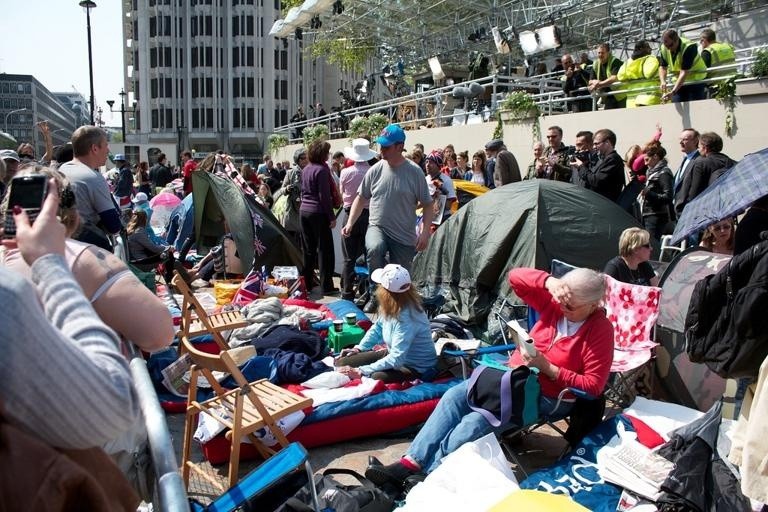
left=321, top=287, right=339, bottom=295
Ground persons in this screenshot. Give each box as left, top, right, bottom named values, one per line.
left=0, top=27, right=768, bottom=512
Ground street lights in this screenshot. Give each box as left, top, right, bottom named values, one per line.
left=4, top=0, right=138, bottom=160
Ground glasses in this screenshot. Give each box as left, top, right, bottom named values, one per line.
left=665, top=42, right=674, bottom=49
left=547, top=135, right=557, bottom=138
left=713, top=225, right=731, bottom=231
left=18, top=152, right=35, bottom=159
left=592, top=138, right=607, bottom=145
left=641, top=242, right=650, bottom=248
left=565, top=303, right=586, bottom=312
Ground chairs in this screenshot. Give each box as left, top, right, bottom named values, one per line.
left=182, top=336, right=313, bottom=493
left=169, top=270, right=248, bottom=354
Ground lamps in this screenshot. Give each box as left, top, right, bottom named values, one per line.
left=535, top=25, right=562, bottom=51
left=519, top=30, right=539, bottom=56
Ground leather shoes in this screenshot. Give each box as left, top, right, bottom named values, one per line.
left=368, top=455, right=383, bottom=465
left=365, top=461, right=413, bottom=496
left=363, top=299, right=377, bottom=313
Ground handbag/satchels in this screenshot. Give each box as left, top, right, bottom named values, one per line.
left=466, top=358, right=541, bottom=428
left=231, top=267, right=262, bottom=307
left=280, top=468, right=395, bottom=512
left=210, top=246, right=224, bottom=275
left=214, top=279, right=243, bottom=306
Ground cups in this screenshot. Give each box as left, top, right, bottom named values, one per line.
left=332, top=320, right=345, bottom=332
left=345, top=312, right=357, bottom=325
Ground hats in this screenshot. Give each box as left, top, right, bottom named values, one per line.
left=131, top=192, right=148, bottom=203
left=485, top=140, right=503, bottom=149
left=425, top=150, right=444, bottom=170
left=376, top=125, right=405, bottom=147
left=0, top=149, right=20, bottom=163
left=344, top=138, right=378, bottom=163
left=113, top=154, right=126, bottom=161
left=371, top=263, right=412, bottom=293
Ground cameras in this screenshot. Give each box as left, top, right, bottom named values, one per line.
left=6, top=173, right=48, bottom=215
left=568, top=150, right=591, bottom=162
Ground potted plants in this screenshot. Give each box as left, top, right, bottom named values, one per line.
left=709, top=42, right=768, bottom=139
left=491, top=90, right=543, bottom=144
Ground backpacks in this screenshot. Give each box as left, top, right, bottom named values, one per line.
left=683, top=232, right=768, bottom=380
left=706, top=155, right=732, bottom=185
left=252, top=325, right=326, bottom=360
